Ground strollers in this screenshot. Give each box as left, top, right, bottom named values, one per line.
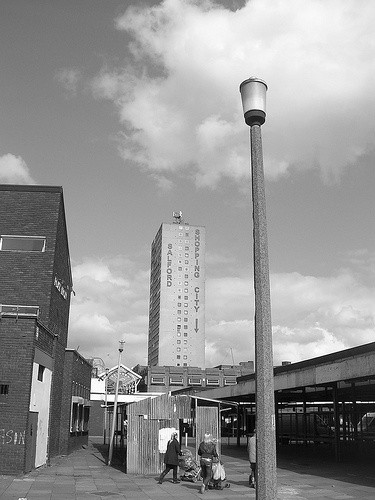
left=208, top=455, right=231, bottom=491
left=177, top=449, right=202, bottom=482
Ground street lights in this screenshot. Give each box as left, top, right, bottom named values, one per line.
left=107, top=340, right=125, bottom=468
left=238, top=76, right=278, bottom=499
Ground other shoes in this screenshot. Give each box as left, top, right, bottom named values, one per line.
left=173, top=481, right=180, bottom=484
left=158, top=477, right=162, bottom=484
left=204, top=489, right=209, bottom=492
left=200, top=484, right=205, bottom=493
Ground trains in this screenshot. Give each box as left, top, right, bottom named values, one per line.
left=331, top=417, right=353, bottom=433
left=221, top=410, right=334, bottom=435
left=354, top=413, right=375, bottom=433
